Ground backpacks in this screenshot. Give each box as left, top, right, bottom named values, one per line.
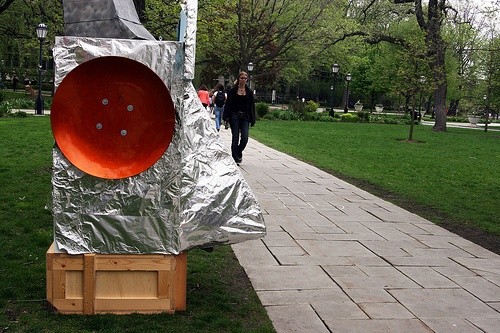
left=215, top=91, right=225, bottom=108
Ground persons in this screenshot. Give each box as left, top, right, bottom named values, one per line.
left=13, top=75, right=20, bottom=91
left=197, top=83, right=232, bottom=132
left=222, top=71, right=256, bottom=165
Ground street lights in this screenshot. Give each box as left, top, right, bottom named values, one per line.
left=344, top=71, right=352, bottom=113
left=35, top=23, right=47, bottom=116
left=420, top=75, right=426, bottom=123
left=247, top=62, right=255, bottom=89
left=329, top=61, right=339, bottom=118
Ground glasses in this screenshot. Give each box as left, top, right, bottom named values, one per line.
left=239, top=77, right=247, bottom=81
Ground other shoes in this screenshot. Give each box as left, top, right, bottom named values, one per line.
left=225, top=125, right=228, bottom=129
left=238, top=157, right=243, bottom=162
left=217, top=129, right=219, bottom=132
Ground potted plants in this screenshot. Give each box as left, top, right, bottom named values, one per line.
left=468, top=113, right=481, bottom=127
left=414, top=107, right=427, bottom=119
left=353, top=104, right=364, bottom=112
left=375, top=103, right=384, bottom=112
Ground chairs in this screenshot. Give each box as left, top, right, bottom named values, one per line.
left=25, top=85, right=34, bottom=94
